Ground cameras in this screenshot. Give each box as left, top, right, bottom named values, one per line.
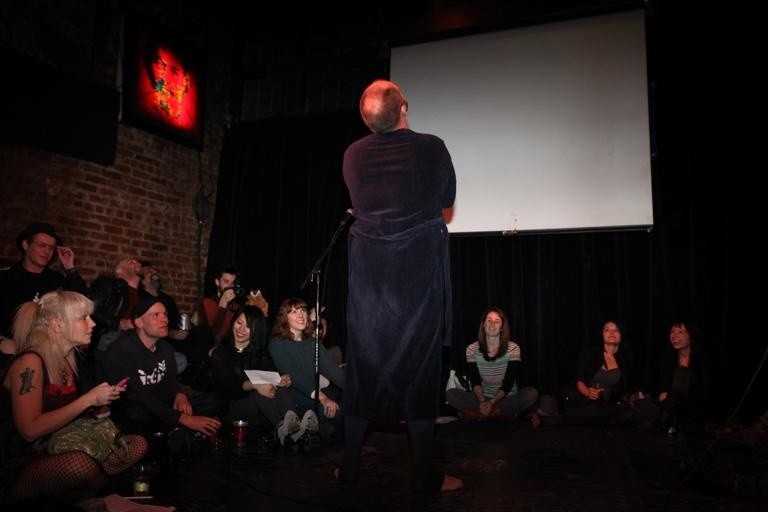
left=225, top=287, right=246, bottom=298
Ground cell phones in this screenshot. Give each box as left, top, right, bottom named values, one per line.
left=114, top=376, right=130, bottom=387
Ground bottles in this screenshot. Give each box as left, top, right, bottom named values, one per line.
left=133, top=464, right=150, bottom=496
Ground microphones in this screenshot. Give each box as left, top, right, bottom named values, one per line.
left=340, top=207, right=353, bottom=225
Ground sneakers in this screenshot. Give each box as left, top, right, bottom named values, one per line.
left=277, top=410, right=318, bottom=455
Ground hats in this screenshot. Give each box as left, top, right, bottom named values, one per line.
left=132, top=295, right=162, bottom=319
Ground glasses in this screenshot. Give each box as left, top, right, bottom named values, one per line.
left=33, top=241, right=56, bottom=250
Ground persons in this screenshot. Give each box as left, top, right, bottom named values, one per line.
left=332, top=79, right=463, bottom=494
left=631, top=318, right=722, bottom=501
left=146, top=34, right=194, bottom=128
left=1, top=224, right=344, bottom=511
left=560, top=319, right=633, bottom=489
left=446, top=307, right=539, bottom=434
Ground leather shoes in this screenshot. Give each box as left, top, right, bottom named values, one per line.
left=461, top=404, right=517, bottom=417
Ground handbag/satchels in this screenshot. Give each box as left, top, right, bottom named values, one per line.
left=47, top=418, right=129, bottom=466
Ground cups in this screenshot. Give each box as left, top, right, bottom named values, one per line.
left=232, top=420, right=249, bottom=447
